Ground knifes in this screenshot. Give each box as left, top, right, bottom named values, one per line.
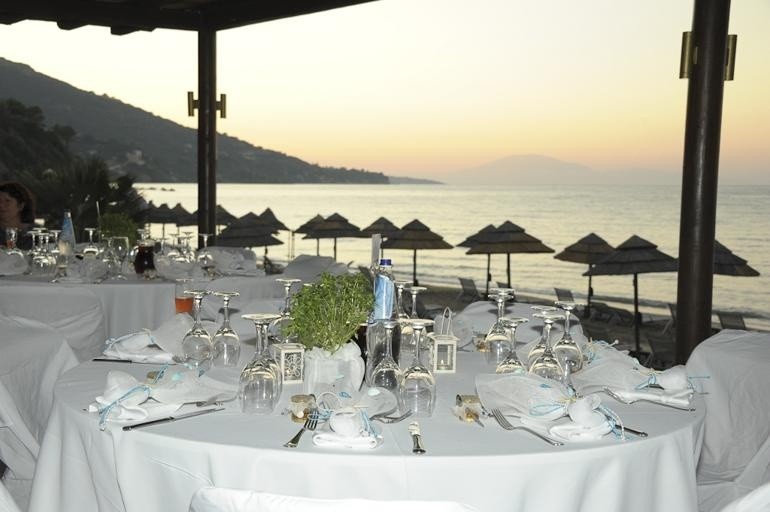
left=92, top=356, right=176, bottom=369
left=123, top=407, right=227, bottom=432
left=410, top=423, right=425, bottom=454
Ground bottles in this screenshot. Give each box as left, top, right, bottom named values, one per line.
left=366, top=258, right=403, bottom=385
left=59, top=208, right=76, bottom=254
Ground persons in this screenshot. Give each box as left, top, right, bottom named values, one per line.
left=0, top=181, right=38, bottom=250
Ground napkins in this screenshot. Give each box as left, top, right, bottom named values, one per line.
left=105, top=312, right=219, bottom=363
left=87, top=367, right=238, bottom=426
left=568, top=339, right=694, bottom=410
left=474, top=371, right=618, bottom=444
left=309, top=387, right=399, bottom=453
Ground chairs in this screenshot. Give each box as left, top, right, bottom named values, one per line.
left=716, top=312, right=748, bottom=327
left=452, top=299, right=584, bottom=368
left=201, top=246, right=258, bottom=276
left=0, top=317, right=81, bottom=512
left=283, top=255, right=335, bottom=278
left=687, top=329, right=766, bottom=510
left=554, top=289, right=636, bottom=324
left=665, top=303, right=679, bottom=334
left=458, top=278, right=515, bottom=302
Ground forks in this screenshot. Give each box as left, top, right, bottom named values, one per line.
left=282, top=407, right=322, bottom=452
left=604, top=388, right=696, bottom=415
left=491, top=408, right=567, bottom=446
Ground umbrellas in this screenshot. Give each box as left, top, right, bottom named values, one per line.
left=713, top=239, right=760, bottom=276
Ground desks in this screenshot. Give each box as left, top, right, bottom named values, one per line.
left=55, top=314, right=706, bottom=511
left=0, top=269, right=318, bottom=356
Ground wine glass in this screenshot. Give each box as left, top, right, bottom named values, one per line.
left=1, top=224, right=218, bottom=282
left=176, top=277, right=585, bottom=425
